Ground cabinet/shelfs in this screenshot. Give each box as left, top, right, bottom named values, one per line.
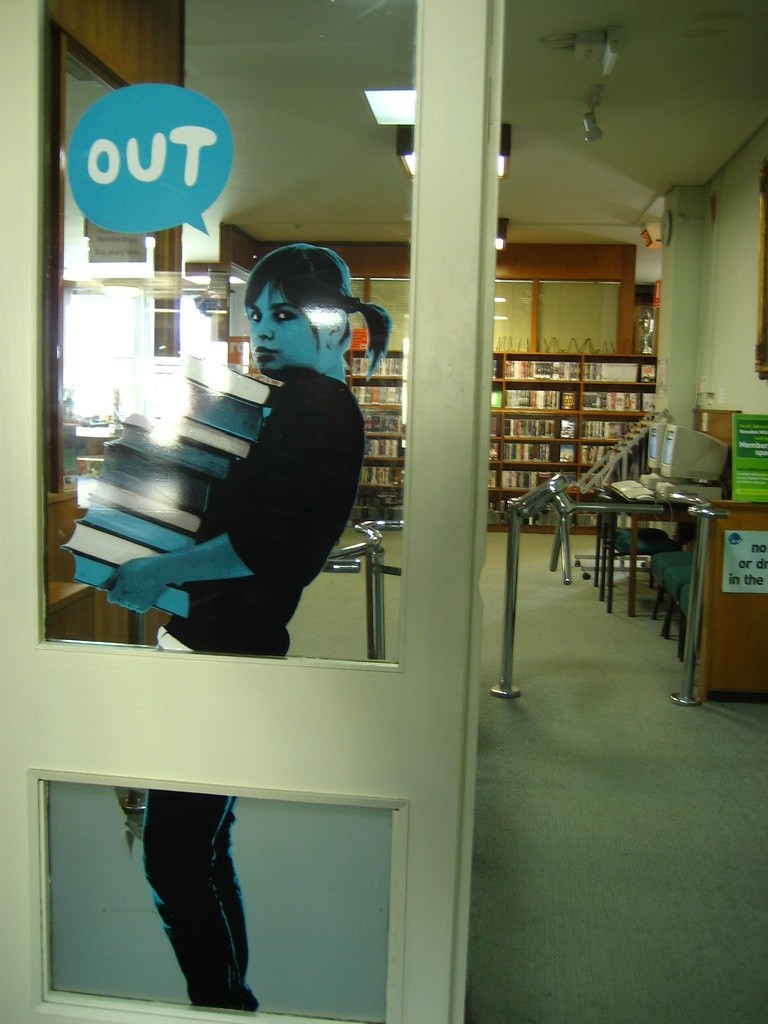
left=342, top=350, right=658, bottom=535
left=44, top=484, right=129, bottom=645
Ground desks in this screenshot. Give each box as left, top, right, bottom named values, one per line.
left=593, top=484, right=704, bottom=617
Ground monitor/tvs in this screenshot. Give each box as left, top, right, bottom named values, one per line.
left=661, top=424, right=728, bottom=484
left=647, top=421, right=669, bottom=469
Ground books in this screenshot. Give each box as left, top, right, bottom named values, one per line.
left=57, top=348, right=275, bottom=619
left=342, top=353, right=659, bottom=527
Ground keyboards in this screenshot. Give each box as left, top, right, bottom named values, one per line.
left=610, top=480, right=643, bottom=494
left=620, top=486, right=655, bottom=501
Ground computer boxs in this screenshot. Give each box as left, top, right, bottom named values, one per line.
left=641, top=473, right=722, bottom=505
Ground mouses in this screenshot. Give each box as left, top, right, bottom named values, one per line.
left=635, top=495, right=655, bottom=501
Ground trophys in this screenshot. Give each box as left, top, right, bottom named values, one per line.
left=638, top=310, right=655, bottom=355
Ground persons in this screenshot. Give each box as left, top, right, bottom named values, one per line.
left=93, top=243, right=391, bottom=1016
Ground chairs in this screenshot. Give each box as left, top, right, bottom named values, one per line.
left=592, top=486, right=670, bottom=602
left=649, top=548, right=700, bottom=665
left=599, top=492, right=682, bottom=615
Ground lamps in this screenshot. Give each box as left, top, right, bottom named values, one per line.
left=582, top=103, right=603, bottom=143
